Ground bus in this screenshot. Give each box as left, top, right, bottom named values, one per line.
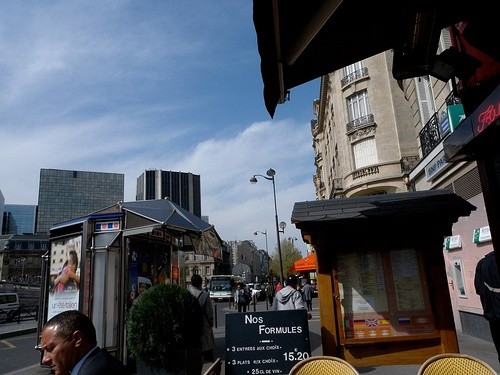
left=208, top=275, right=239, bottom=302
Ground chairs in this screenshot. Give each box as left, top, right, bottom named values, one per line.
left=288, top=355, right=359, bottom=375
left=417, top=353, right=497, bottom=375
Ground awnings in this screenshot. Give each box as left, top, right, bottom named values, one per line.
left=253, top=0, right=414, bottom=118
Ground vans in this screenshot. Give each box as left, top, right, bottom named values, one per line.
left=0, top=293, right=19, bottom=314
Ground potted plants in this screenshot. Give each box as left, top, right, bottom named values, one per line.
left=126, top=284, right=206, bottom=374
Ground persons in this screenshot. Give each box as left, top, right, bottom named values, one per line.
left=266, top=280, right=281, bottom=306
left=188, top=274, right=216, bottom=363
left=272, top=274, right=312, bottom=320
left=50, top=250, right=80, bottom=297
left=42, top=310, right=131, bottom=375
left=474, top=252, right=500, bottom=362
left=234, top=282, right=249, bottom=312
left=297, top=280, right=312, bottom=311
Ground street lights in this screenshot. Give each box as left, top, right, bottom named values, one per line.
left=253, top=229, right=271, bottom=285
left=249, top=168, right=286, bottom=288
left=288, top=236, right=297, bottom=249
